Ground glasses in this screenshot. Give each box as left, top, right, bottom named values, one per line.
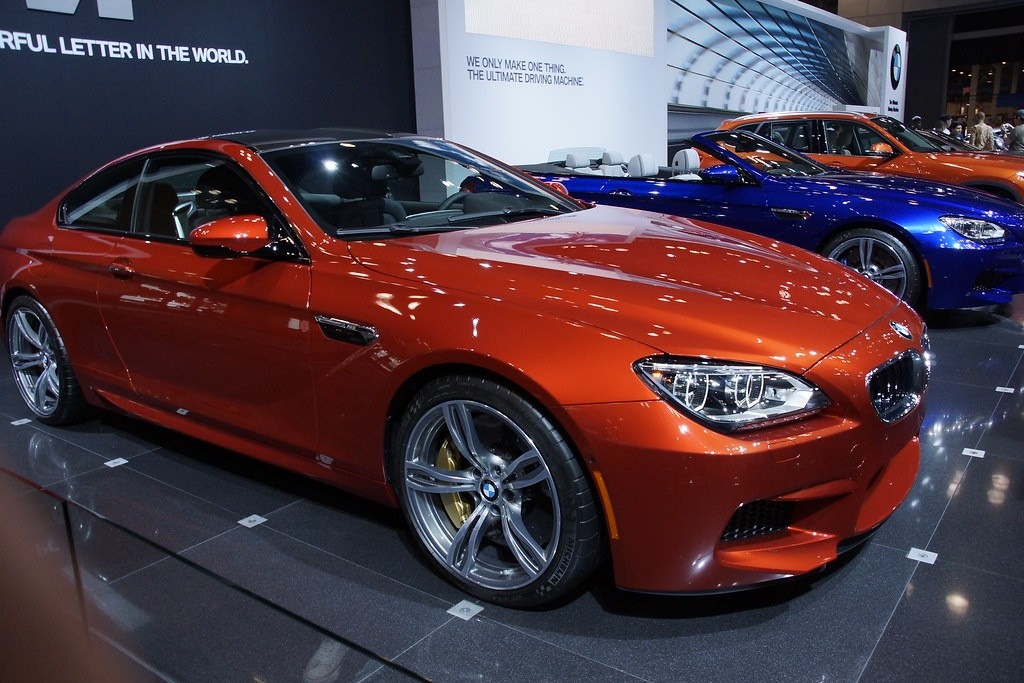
left=1014, top=115, right=1022, bottom=119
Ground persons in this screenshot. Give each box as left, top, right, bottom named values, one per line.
left=912, top=109, right=1024, bottom=152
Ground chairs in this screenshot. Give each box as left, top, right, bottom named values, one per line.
left=190, top=166, right=241, bottom=228
left=793, top=134, right=808, bottom=149
left=599, top=150, right=626, bottom=177
left=668, top=149, right=702, bottom=181
left=835, top=131, right=854, bottom=155
left=827, top=127, right=838, bottom=154
left=625, top=151, right=653, bottom=177
left=329, top=162, right=406, bottom=229
left=564, top=152, right=594, bottom=174
left=118, top=182, right=191, bottom=239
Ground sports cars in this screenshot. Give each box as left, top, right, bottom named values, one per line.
left=461, top=131, right=1023, bottom=319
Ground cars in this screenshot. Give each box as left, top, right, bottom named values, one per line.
left=801, top=128, right=980, bottom=151
left=0, top=127, right=934, bottom=614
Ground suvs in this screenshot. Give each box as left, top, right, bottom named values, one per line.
left=689, top=112, right=1024, bottom=204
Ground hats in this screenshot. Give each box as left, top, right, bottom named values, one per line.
left=1017, top=109, right=1024, bottom=118
left=912, top=114, right=921, bottom=121
